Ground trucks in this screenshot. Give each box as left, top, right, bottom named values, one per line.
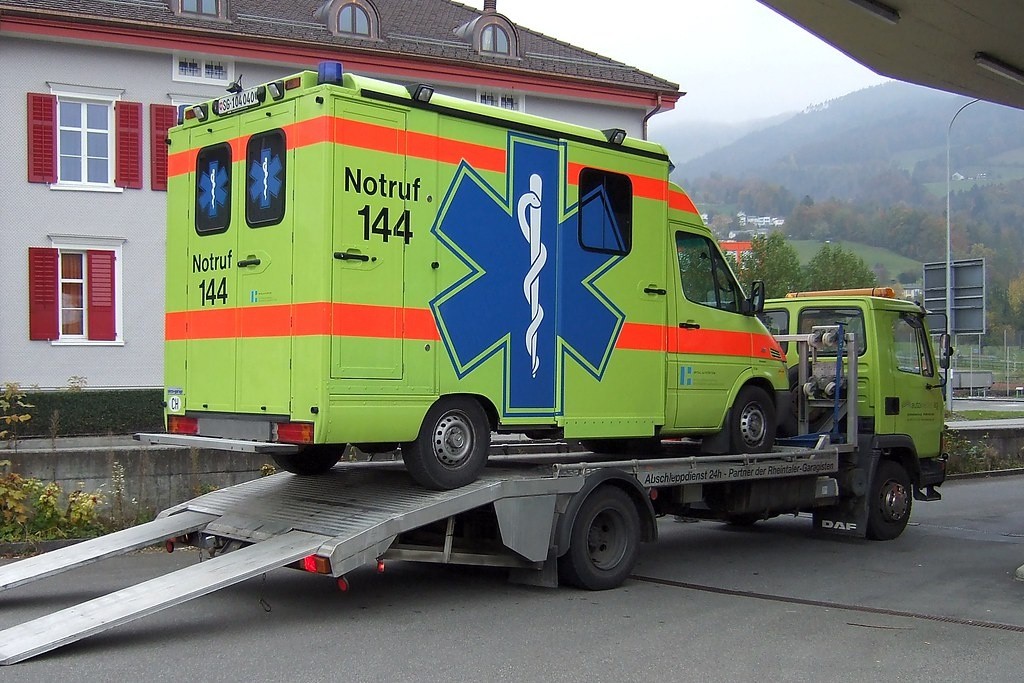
left=0, top=284, right=958, bottom=667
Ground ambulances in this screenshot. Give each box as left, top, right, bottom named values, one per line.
left=132, top=60, right=795, bottom=495
